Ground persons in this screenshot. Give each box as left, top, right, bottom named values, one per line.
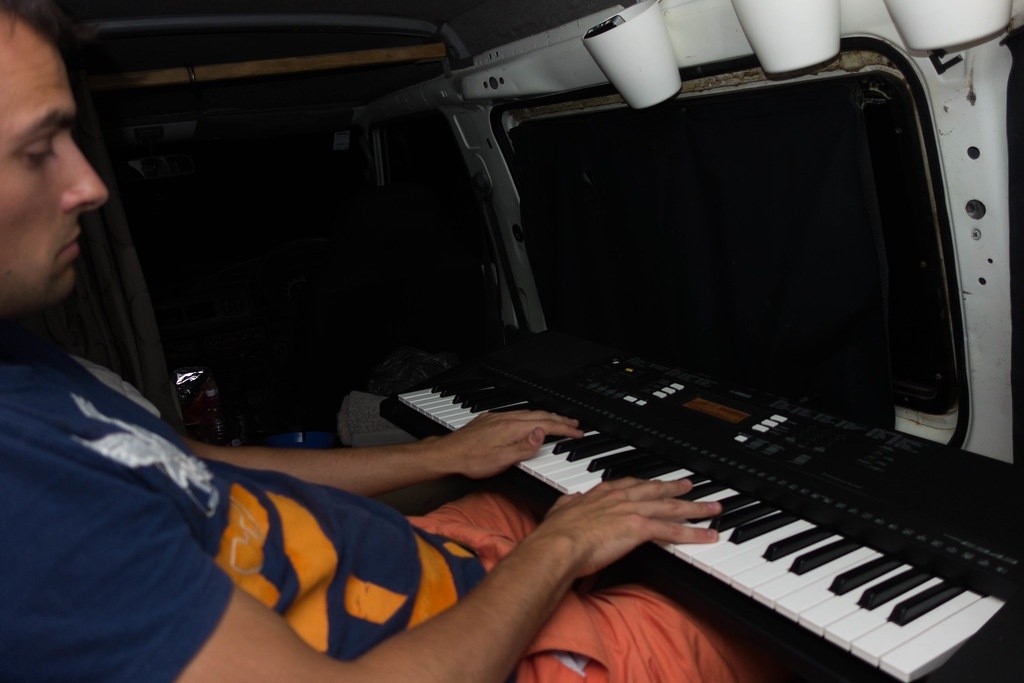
left=1, top=0, right=771, bottom=682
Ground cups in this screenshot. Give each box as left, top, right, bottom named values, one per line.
left=730, top=1, right=840, bottom=74
left=883, top=0, right=1013, bottom=51
left=582, top=1, right=682, bottom=110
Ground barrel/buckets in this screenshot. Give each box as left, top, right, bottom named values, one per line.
left=264, top=431, right=336, bottom=450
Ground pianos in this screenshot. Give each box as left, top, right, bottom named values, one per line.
left=378, top=328, right=1024, bottom=683
